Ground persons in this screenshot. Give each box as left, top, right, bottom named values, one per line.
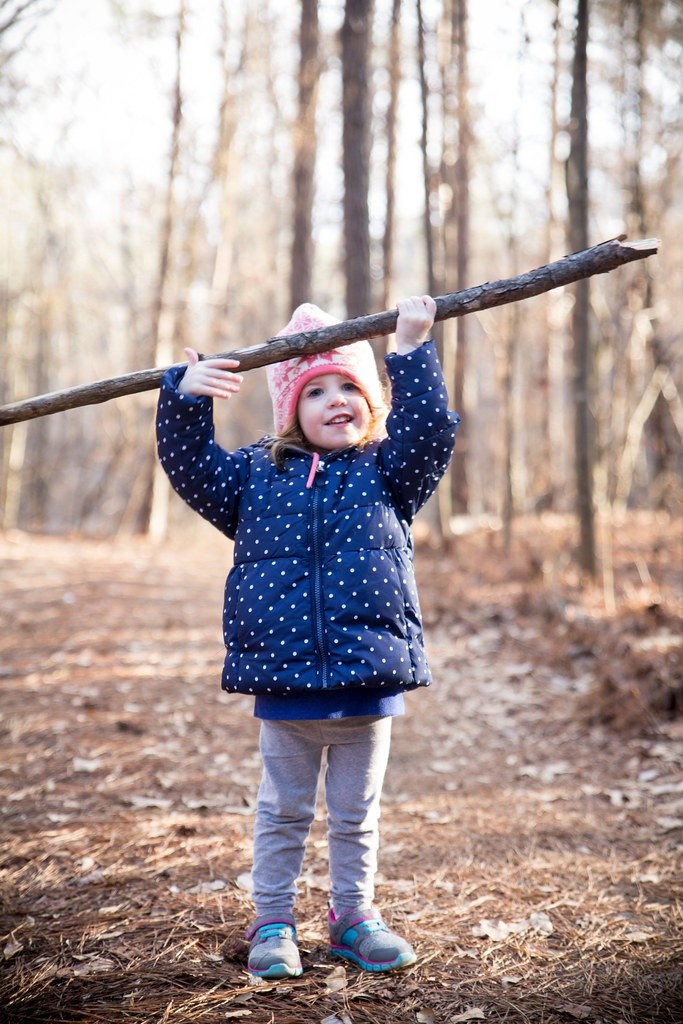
left=155, top=295, right=461, bottom=977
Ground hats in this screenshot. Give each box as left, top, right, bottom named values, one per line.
left=266, top=303, right=386, bottom=432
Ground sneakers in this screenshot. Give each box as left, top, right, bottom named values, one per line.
left=245, top=912, right=304, bottom=979
left=326, top=901, right=416, bottom=972
